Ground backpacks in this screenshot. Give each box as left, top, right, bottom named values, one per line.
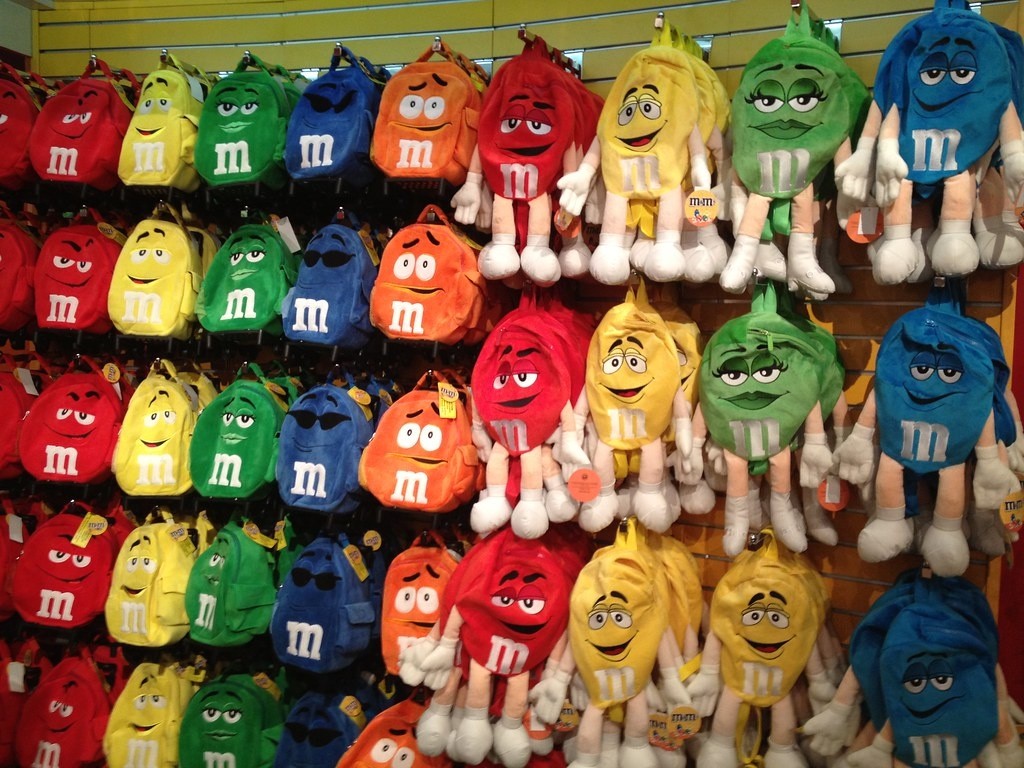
left=0, top=0, right=1024, bottom=768
